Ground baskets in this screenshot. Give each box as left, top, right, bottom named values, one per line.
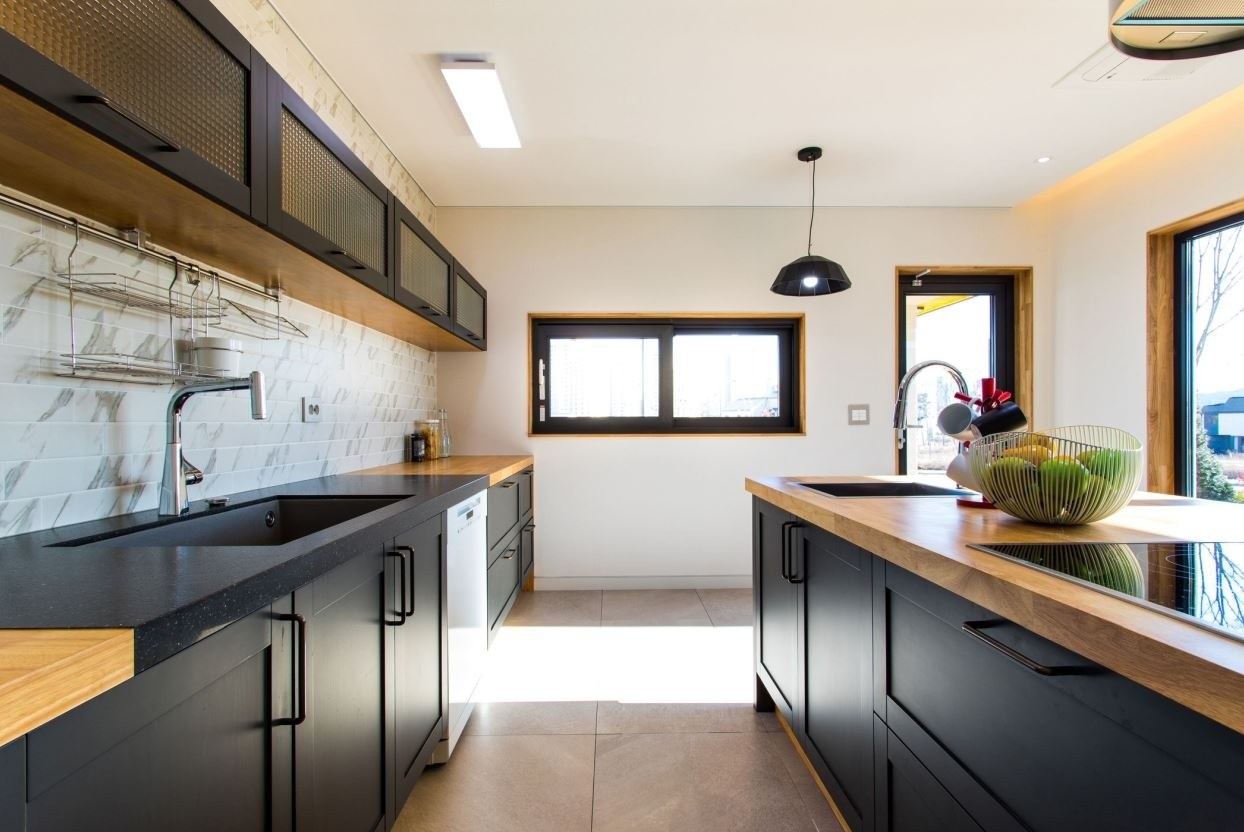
left=969, top=423, right=1143, bottom=524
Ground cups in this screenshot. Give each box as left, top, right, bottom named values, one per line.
left=937, top=397, right=983, bottom=442
left=970, top=399, right=1028, bottom=445
left=946, top=443, right=983, bottom=494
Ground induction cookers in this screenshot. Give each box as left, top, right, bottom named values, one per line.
left=965, top=541, right=1244, bottom=642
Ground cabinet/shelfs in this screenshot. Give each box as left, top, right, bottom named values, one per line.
left=752, top=494, right=872, bottom=832
left=57, top=217, right=231, bottom=387
left=292, top=511, right=448, bottom=832
left=487, top=464, right=534, bottom=651
left=0, top=0, right=488, bottom=353
left=0, top=593, right=293, bottom=832
left=872, top=554, right=1244, bottom=832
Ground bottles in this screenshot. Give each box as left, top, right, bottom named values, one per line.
left=411, top=408, right=451, bottom=462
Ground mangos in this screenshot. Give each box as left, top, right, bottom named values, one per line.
left=990, top=545, right=1136, bottom=594
left=983, top=431, right=1128, bottom=524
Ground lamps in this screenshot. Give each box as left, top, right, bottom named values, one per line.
left=439, top=62, right=522, bottom=149
left=769, top=147, right=852, bottom=297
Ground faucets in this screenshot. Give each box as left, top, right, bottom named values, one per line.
left=893, top=360, right=972, bottom=489
left=158, top=369, right=267, bottom=516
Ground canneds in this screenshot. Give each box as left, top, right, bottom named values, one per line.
left=409, top=433, right=425, bottom=462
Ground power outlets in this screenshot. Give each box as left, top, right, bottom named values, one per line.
left=302, top=398, right=322, bottom=423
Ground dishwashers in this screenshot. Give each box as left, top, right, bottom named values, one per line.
left=425, top=488, right=488, bottom=768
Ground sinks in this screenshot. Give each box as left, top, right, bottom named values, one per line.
left=786, top=481, right=981, bottom=499
left=41, top=494, right=417, bottom=546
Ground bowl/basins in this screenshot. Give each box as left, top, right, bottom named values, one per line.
left=193, top=336, right=243, bottom=376
